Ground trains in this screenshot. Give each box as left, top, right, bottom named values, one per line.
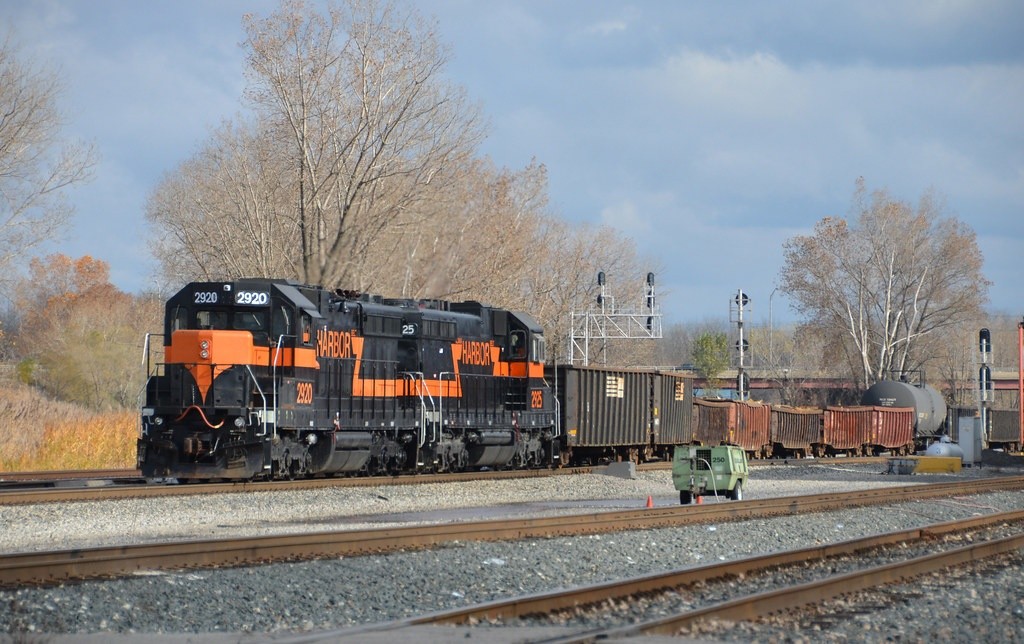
left=135, top=278, right=1024, bottom=484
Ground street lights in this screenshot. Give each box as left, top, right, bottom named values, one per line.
left=769, top=288, right=780, bottom=371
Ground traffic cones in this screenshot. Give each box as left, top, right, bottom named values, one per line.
left=694, top=495, right=704, bottom=505
left=646, top=495, right=654, bottom=509
